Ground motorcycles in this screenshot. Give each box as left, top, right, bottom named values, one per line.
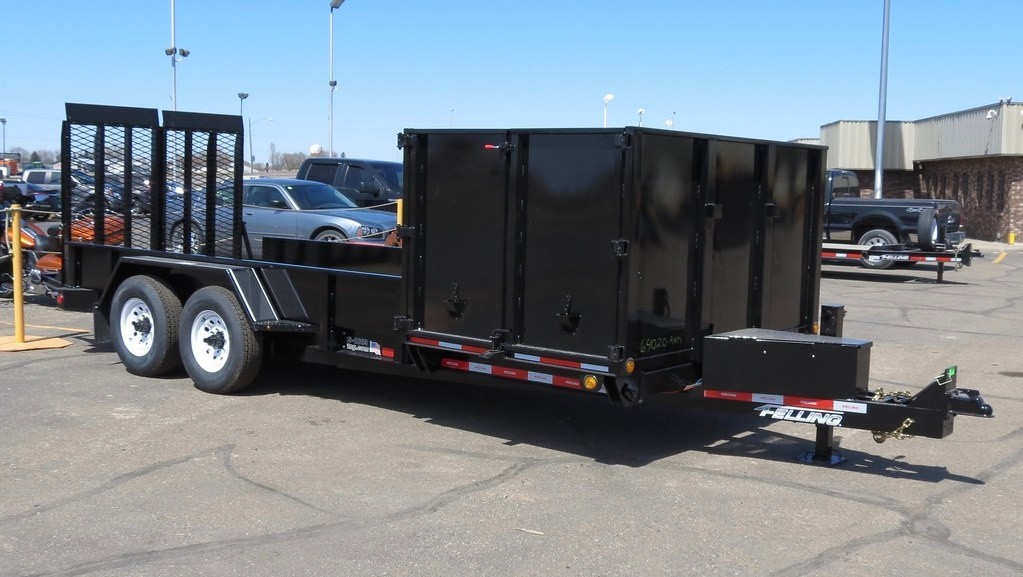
left=0, top=214, right=127, bottom=310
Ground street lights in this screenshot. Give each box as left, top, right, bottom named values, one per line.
left=165, top=0, right=190, bottom=191
left=328, top=0, right=346, bottom=159
left=601, top=93, right=614, bottom=128
left=237, top=91, right=249, bottom=117
left=0, top=118, right=8, bottom=157
left=248, top=114, right=274, bottom=176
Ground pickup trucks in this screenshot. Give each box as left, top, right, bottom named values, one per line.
left=825, top=168, right=966, bottom=267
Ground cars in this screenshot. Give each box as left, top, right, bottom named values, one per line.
left=161, top=177, right=397, bottom=261
left=0, top=178, right=59, bottom=223
left=103, top=173, right=171, bottom=214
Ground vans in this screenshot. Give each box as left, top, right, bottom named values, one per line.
left=21, top=169, right=123, bottom=214
left=299, top=158, right=404, bottom=216
